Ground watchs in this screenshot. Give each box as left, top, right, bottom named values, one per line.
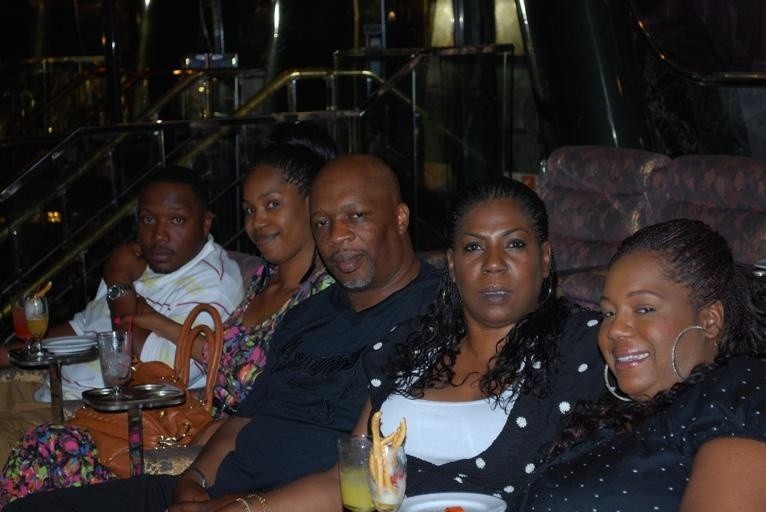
left=106, top=282, right=141, bottom=302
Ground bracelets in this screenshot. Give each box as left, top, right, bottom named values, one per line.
left=230, top=490, right=271, bottom=512
left=182, top=464, right=211, bottom=490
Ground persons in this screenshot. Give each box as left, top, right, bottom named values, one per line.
left=1, top=153, right=474, bottom=512
left=486, top=216, right=765, bottom=512
left=2, top=140, right=330, bottom=512
left=200, top=174, right=609, bottom=512
left=0, top=166, right=246, bottom=402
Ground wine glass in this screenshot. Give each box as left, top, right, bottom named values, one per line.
left=11, top=293, right=51, bottom=360
left=98, top=329, right=134, bottom=401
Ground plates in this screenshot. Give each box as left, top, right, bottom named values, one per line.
left=401, top=491, right=510, bottom=512
left=37, top=336, right=98, bottom=354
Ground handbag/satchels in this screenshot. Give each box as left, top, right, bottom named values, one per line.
left=69, top=303, right=224, bottom=481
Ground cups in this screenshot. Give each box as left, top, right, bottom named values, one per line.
left=366, top=444, right=409, bottom=512
left=336, top=433, right=377, bottom=511
